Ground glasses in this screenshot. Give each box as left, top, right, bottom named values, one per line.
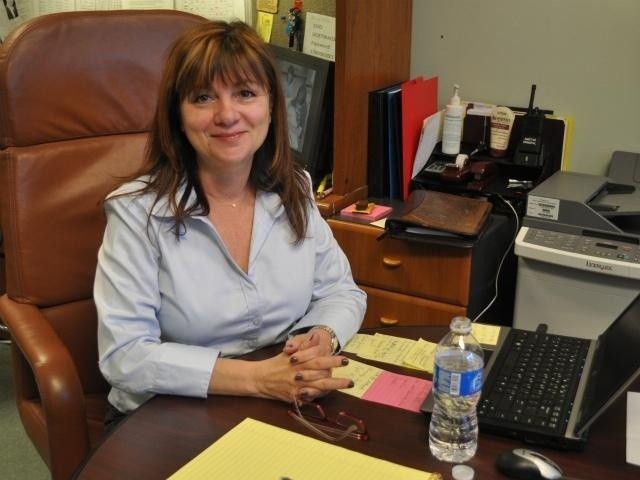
left=285, top=393, right=371, bottom=445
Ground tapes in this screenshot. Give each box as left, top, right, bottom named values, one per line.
left=456, top=154, right=469, bottom=169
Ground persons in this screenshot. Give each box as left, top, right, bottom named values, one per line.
left=91, top=17, right=373, bottom=443
left=291, top=86, right=307, bottom=128
left=281, top=63, right=300, bottom=100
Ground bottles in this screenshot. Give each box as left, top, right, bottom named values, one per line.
left=427, top=314, right=486, bottom=463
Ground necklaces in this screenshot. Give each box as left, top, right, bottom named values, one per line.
left=200, top=183, right=257, bottom=211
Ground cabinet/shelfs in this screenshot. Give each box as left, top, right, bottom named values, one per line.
left=327, top=220, right=473, bottom=333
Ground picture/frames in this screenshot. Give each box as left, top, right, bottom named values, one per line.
left=262, top=42, right=334, bottom=178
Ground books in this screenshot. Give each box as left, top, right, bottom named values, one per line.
left=385, top=71, right=440, bottom=204
left=381, top=186, right=498, bottom=252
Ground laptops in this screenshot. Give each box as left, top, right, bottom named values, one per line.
left=419, top=294, right=640, bottom=452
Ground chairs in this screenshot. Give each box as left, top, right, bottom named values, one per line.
left=0, top=7, right=331, bottom=480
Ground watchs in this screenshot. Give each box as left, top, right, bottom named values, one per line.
left=312, top=323, right=341, bottom=357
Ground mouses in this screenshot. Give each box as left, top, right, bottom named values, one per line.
left=495, top=447, right=564, bottom=479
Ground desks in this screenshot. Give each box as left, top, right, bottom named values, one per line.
left=64, top=322, right=639, bottom=479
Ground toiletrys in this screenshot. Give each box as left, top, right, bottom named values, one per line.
left=489, top=104, right=517, bottom=158
left=490, top=107, right=515, bottom=156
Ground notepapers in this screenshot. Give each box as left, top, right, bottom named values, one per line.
left=340, top=201, right=392, bottom=221
